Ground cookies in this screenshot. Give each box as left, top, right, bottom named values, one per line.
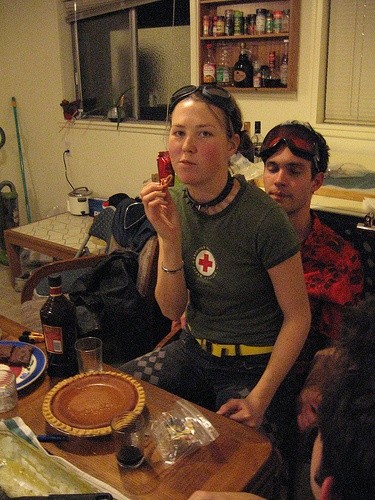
left=160, top=174, right=173, bottom=186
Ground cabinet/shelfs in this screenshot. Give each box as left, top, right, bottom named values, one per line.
left=197, top=0, right=301, bottom=93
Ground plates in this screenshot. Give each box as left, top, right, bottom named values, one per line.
left=41, top=371, right=146, bottom=439
left=0, top=340, right=47, bottom=392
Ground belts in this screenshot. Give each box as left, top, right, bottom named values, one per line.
left=194, top=338, right=274, bottom=357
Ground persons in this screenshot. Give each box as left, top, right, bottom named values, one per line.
left=118, top=85, right=312, bottom=495
left=177, top=119, right=367, bottom=462
left=185, top=288, right=375, bottom=500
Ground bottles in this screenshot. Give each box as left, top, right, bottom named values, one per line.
left=255, top=8, right=266, bottom=35
left=253, top=60, right=262, bottom=88
left=233, top=42, right=253, bottom=87
left=244, top=14, right=256, bottom=36
left=244, top=122, right=251, bottom=138
left=202, top=15, right=224, bottom=37
left=224, top=9, right=244, bottom=36
left=279, top=40, right=289, bottom=88
left=39, top=273, right=79, bottom=377
left=217, top=43, right=234, bottom=86
left=203, top=43, right=217, bottom=87
left=251, top=120, right=265, bottom=163
left=265, top=8, right=289, bottom=34
left=263, top=50, right=280, bottom=87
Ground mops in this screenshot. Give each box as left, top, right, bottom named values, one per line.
left=11, top=95, right=42, bottom=269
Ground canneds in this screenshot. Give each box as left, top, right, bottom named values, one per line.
left=202, top=8, right=284, bottom=37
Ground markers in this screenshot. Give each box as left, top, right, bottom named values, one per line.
left=36, top=435, right=68, bottom=443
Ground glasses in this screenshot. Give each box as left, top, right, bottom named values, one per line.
left=258, top=125, right=321, bottom=161
left=168, top=85, right=236, bottom=113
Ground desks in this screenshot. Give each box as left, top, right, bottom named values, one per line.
left=0, top=315, right=284, bottom=500
left=4, top=212, right=108, bottom=290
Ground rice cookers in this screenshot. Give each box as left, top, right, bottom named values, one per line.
left=67, top=186, right=93, bottom=216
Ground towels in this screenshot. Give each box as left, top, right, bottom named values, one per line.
left=0, top=415, right=132, bottom=500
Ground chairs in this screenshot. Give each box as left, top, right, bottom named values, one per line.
left=30, top=206, right=117, bottom=298
left=19, top=198, right=158, bottom=304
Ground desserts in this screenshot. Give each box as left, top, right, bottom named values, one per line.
left=0, top=343, right=34, bottom=367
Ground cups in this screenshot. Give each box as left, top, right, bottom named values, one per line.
left=0, top=370, right=20, bottom=421
left=110, top=410, right=147, bottom=470
left=74, top=336, right=104, bottom=373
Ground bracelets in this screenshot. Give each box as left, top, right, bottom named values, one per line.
left=161, top=258, right=186, bottom=273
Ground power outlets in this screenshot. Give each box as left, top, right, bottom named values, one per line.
left=64, top=140, right=73, bottom=157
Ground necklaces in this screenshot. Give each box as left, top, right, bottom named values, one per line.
left=181, top=169, right=234, bottom=210
